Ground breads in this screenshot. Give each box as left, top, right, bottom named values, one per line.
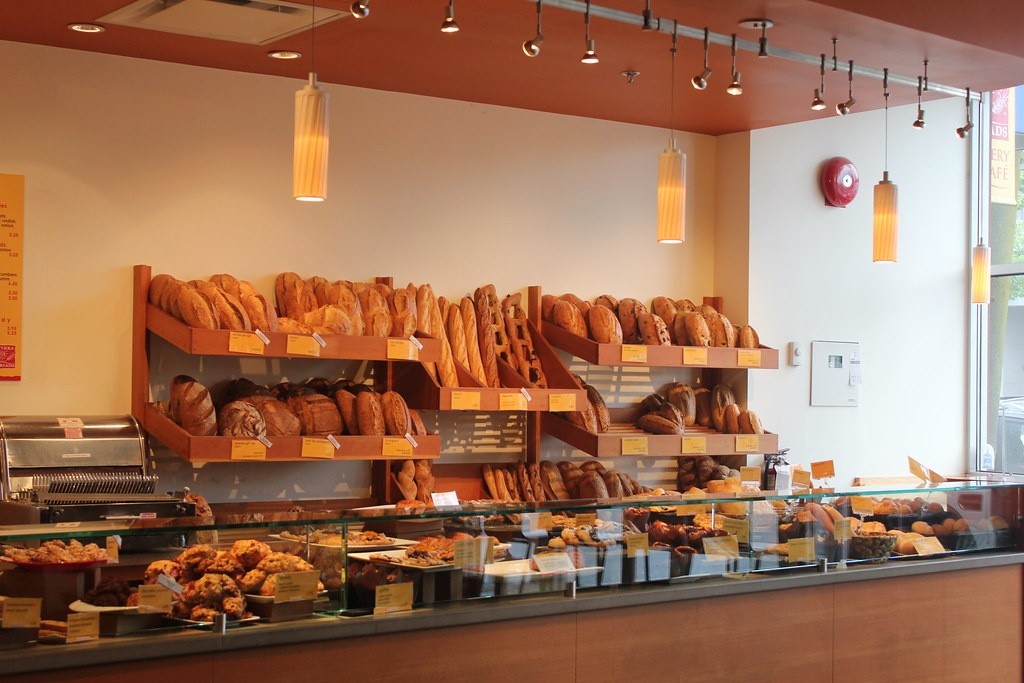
left=148, top=273, right=417, bottom=340
left=0, top=539, right=107, bottom=564
left=278, top=530, right=506, bottom=590
left=482, top=460, right=1008, bottom=563
left=39, top=540, right=322, bottom=639
left=540, top=292, right=760, bottom=348
left=563, top=372, right=764, bottom=435
left=155, top=375, right=434, bottom=508
left=406, top=282, right=550, bottom=388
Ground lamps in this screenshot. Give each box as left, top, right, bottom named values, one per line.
left=873, top=68, right=900, bottom=263
left=656, top=18, right=686, bottom=244
left=973, top=92, right=991, bottom=304
left=350, top=0, right=973, bottom=139
left=293, top=0, right=331, bottom=203
